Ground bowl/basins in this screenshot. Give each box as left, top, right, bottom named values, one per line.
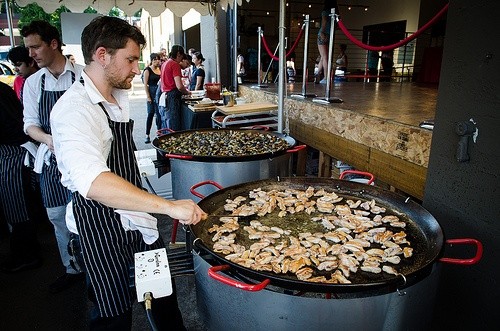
left=201, top=98, right=211, bottom=103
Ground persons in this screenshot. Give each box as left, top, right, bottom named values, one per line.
left=48, top=16, right=208, bottom=331
left=20, top=18, right=86, bottom=295
left=0, top=81, right=41, bottom=271
left=158, top=44, right=192, bottom=135
left=143, top=53, right=162, bottom=144
left=316, top=0, right=341, bottom=85
left=236, top=38, right=349, bottom=85
left=66, top=54, right=76, bottom=63
left=140, top=47, right=206, bottom=134
left=5, top=45, right=41, bottom=101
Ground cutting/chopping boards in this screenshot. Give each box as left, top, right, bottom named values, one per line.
left=215, top=102, right=278, bottom=115
left=188, top=104, right=218, bottom=112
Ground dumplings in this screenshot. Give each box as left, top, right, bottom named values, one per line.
left=209, top=187, right=414, bottom=284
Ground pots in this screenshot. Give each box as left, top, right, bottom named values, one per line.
left=171, top=171, right=482, bottom=292
left=151, top=128, right=307, bottom=163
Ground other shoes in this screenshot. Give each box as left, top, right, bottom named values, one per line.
left=145, top=137, right=150, bottom=143
left=48, top=273, right=83, bottom=295
left=315, top=69, right=321, bottom=76
left=319, top=78, right=329, bottom=84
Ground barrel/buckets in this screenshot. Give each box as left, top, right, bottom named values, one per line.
left=222, top=92, right=234, bottom=107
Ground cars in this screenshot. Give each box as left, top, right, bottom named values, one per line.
left=0, top=51, right=9, bottom=62
left=0, top=61, right=17, bottom=90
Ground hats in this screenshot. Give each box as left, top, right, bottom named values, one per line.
left=171, top=44, right=188, bottom=59
left=184, top=55, right=195, bottom=66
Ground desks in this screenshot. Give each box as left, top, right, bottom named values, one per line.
left=180, top=90, right=224, bottom=131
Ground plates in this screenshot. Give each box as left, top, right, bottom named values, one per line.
left=197, top=100, right=218, bottom=105
